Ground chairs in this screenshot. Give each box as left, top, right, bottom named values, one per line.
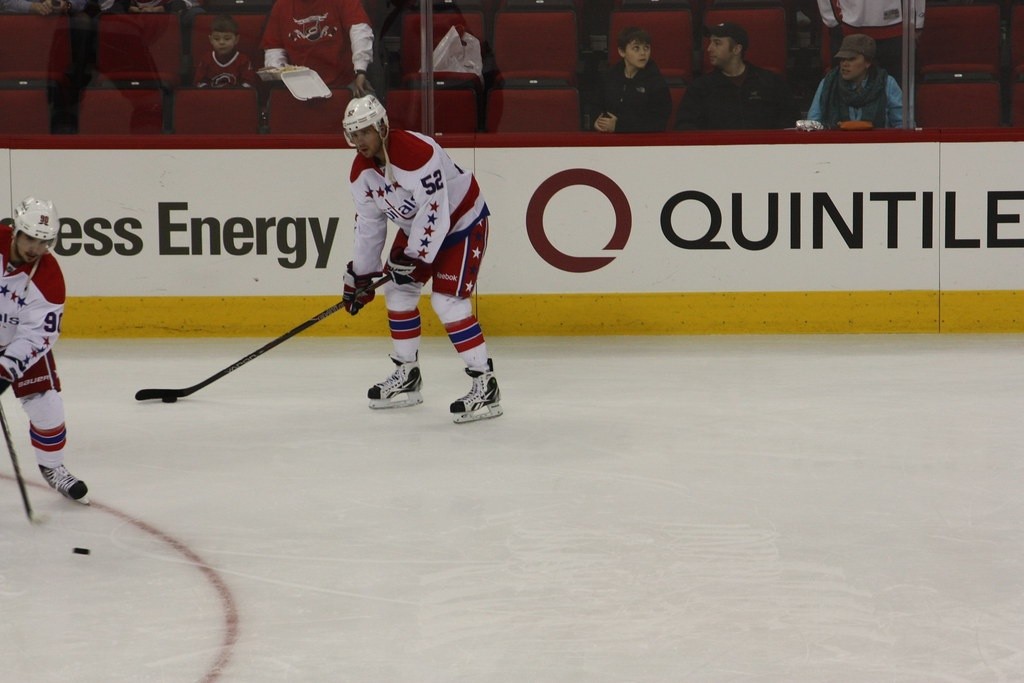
left=0, top=0, right=1024, bottom=146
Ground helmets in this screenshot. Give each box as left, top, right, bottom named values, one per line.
left=12, top=198, right=60, bottom=253
left=342, top=94, right=389, bottom=147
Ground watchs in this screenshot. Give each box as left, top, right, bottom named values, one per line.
left=353, top=69, right=365, bottom=76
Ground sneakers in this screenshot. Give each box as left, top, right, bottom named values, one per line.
left=38, top=464, right=89, bottom=500
left=450, top=358, right=501, bottom=413
left=368, top=349, right=423, bottom=399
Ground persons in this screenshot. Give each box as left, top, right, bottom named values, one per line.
left=815, top=0, right=925, bottom=117
left=0, top=197, right=88, bottom=506
left=0, top=0, right=375, bottom=98
left=677, top=23, right=802, bottom=129
left=590, top=26, right=673, bottom=133
left=806, top=33, right=903, bottom=128
left=343, top=95, right=503, bottom=423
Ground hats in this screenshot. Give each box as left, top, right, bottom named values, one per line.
left=833, top=34, right=876, bottom=59
left=703, top=22, right=748, bottom=51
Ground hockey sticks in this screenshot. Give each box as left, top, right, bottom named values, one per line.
left=134, top=275, right=389, bottom=401
left=0, top=403, right=35, bottom=526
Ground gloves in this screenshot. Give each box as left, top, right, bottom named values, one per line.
left=386, top=248, right=432, bottom=292
left=343, top=262, right=376, bottom=315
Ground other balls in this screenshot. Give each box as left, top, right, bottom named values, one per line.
left=73, top=547, right=90, bottom=555
left=162, top=395, right=177, bottom=402
